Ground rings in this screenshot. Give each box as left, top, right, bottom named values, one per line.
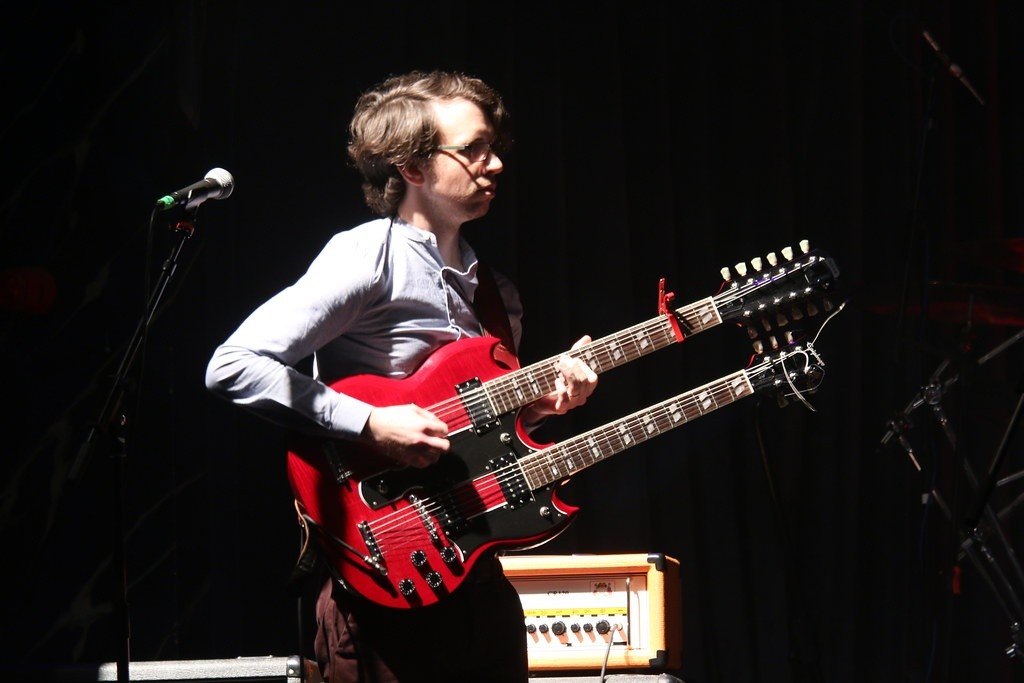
left=567, top=388, right=580, bottom=397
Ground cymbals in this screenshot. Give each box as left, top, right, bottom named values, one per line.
left=941, top=236, right=1024, bottom=273
left=863, top=279, right=1024, bottom=327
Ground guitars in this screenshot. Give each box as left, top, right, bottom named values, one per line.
left=283, top=239, right=848, bottom=609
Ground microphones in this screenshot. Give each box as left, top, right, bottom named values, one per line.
left=921, top=28, right=988, bottom=107
left=157, top=168, right=236, bottom=211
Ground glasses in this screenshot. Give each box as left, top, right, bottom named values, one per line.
left=436, top=138, right=517, bottom=162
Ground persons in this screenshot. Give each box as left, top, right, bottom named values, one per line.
left=205, top=72, right=598, bottom=683
